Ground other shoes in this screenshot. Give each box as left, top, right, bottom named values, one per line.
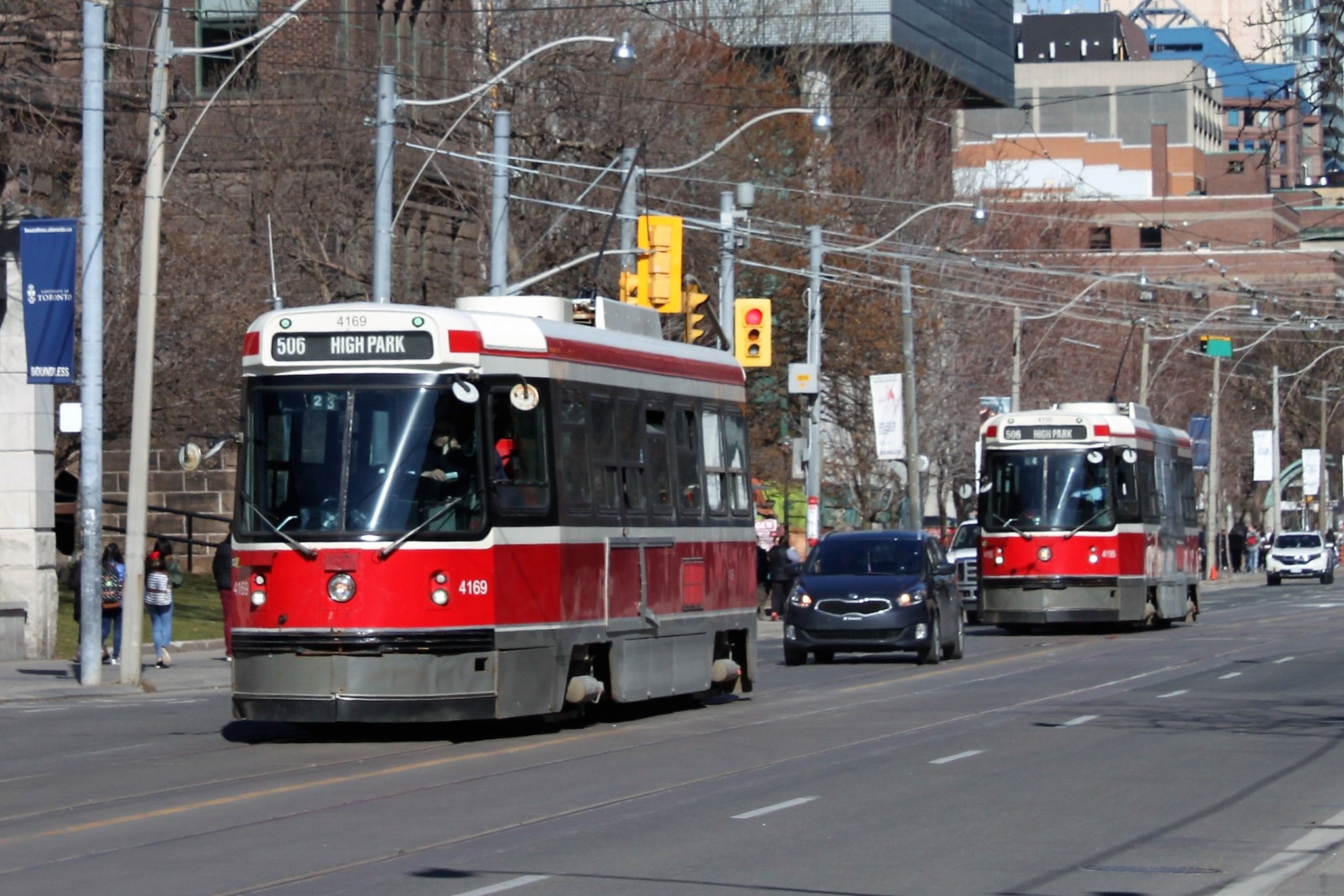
left=102, top=655, right=111, bottom=663
left=111, top=659, right=120, bottom=664
left=771, top=612, right=778, bottom=621
left=160, top=646, right=171, bottom=666
left=155, top=661, right=163, bottom=668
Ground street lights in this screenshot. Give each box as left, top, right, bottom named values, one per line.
left=1010, top=266, right=1151, bottom=413
left=1205, top=307, right=1303, bottom=582
left=373, top=22, right=640, bottom=305
left=1139, top=300, right=1261, bottom=406
left=804, top=202, right=987, bottom=548
left=619, top=105, right=834, bottom=281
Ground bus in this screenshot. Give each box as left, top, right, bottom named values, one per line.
left=960, top=316, right=1201, bottom=632
left=176, top=133, right=759, bottom=735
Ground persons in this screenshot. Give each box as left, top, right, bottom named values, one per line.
left=407, top=422, right=470, bottom=504
left=145, top=537, right=183, bottom=667
left=69, top=549, right=83, bottom=663
left=100, top=542, right=125, bottom=664
left=1326, top=522, right=1344, bottom=568
left=1198, top=523, right=1274, bottom=571
left=755, top=535, right=798, bottom=621
left=1071, top=464, right=1117, bottom=502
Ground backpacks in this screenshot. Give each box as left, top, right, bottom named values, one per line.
left=145, top=561, right=174, bottom=606
left=102, top=563, right=121, bottom=602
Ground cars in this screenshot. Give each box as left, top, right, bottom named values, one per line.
left=783, top=530, right=965, bottom=666
left=1265, top=531, right=1336, bottom=586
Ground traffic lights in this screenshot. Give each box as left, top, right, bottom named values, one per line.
left=733, top=298, right=773, bottom=368
left=683, top=283, right=710, bottom=346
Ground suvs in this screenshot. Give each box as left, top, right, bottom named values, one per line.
left=941, top=520, right=978, bottom=623
